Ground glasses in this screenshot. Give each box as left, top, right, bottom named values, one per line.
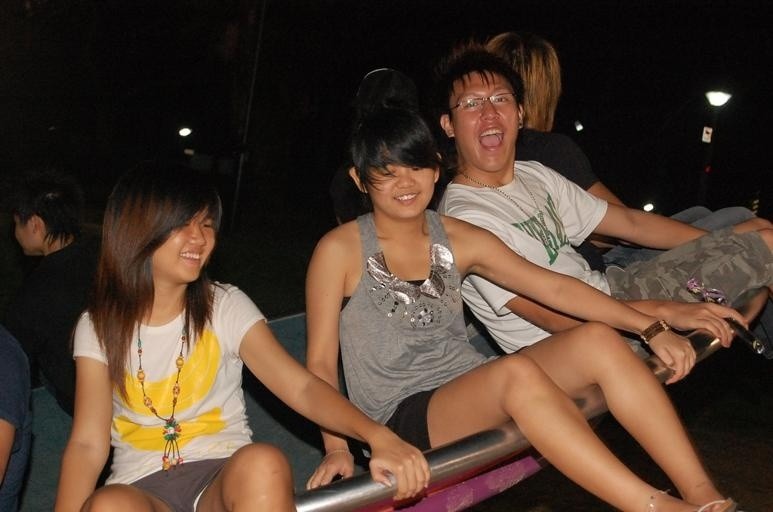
left=450, top=93, right=516, bottom=111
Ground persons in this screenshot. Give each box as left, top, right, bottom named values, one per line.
left=51, top=159, right=432, bottom=512
left=296, top=113, right=749, bottom=512
left=428, top=42, right=773, bottom=378
left=0, top=330, right=32, bottom=511
left=327, top=70, right=449, bottom=225
left=4, top=174, right=100, bottom=415
left=484, top=31, right=757, bottom=271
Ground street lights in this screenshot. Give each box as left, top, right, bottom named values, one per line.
left=692, top=86, right=731, bottom=206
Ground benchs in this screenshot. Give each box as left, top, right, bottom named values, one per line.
left=22, top=313, right=728, bottom=511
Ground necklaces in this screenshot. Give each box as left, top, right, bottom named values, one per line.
left=133, top=308, right=196, bottom=471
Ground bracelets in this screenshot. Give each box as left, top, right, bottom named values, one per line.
left=322, top=450, right=353, bottom=459
left=640, top=320, right=669, bottom=344
